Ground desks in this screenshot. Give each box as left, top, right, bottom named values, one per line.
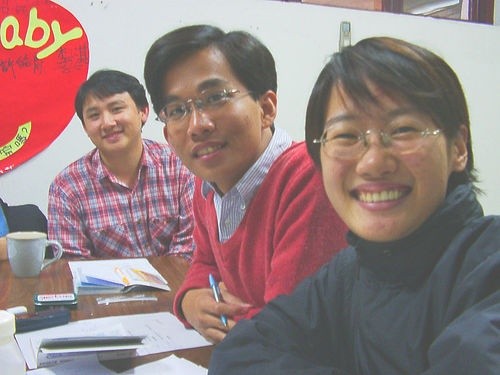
left=0, top=255, right=216, bottom=375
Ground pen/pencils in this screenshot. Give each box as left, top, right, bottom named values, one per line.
left=114, top=266, right=128, bottom=284
left=209, top=274, right=229, bottom=331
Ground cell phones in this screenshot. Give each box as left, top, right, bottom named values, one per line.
left=32, top=292, right=79, bottom=308
left=12, top=305, right=70, bottom=335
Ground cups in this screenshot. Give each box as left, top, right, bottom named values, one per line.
left=6, top=231, right=63, bottom=277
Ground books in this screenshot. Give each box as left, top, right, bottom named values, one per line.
left=29, top=333, right=147, bottom=368
left=74, top=267, right=172, bottom=295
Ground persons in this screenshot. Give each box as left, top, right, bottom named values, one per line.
left=203, top=37, right=500, bottom=375
left=48, top=71, right=195, bottom=263
left=143, top=24, right=351, bottom=343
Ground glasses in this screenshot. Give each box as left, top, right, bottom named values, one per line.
left=313, top=119, right=443, bottom=159
left=154, top=89, right=254, bottom=123
